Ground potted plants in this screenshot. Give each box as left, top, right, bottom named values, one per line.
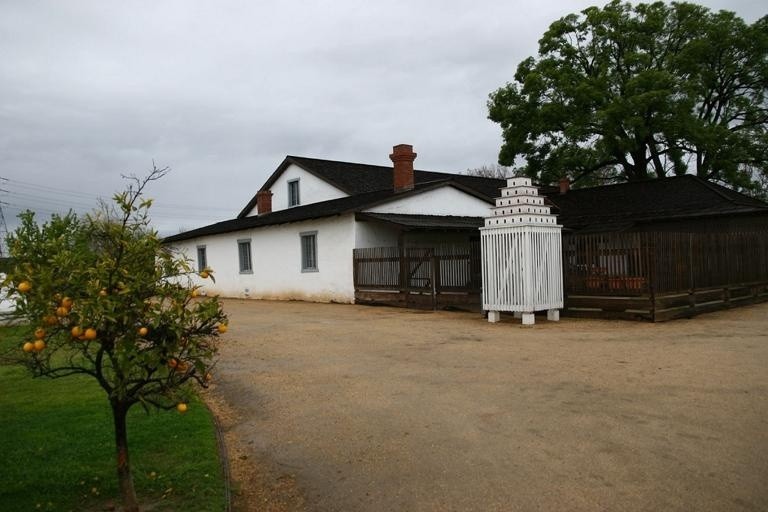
left=583, top=267, right=647, bottom=292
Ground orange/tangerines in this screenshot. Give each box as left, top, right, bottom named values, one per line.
left=18, top=272, right=227, bottom=411
